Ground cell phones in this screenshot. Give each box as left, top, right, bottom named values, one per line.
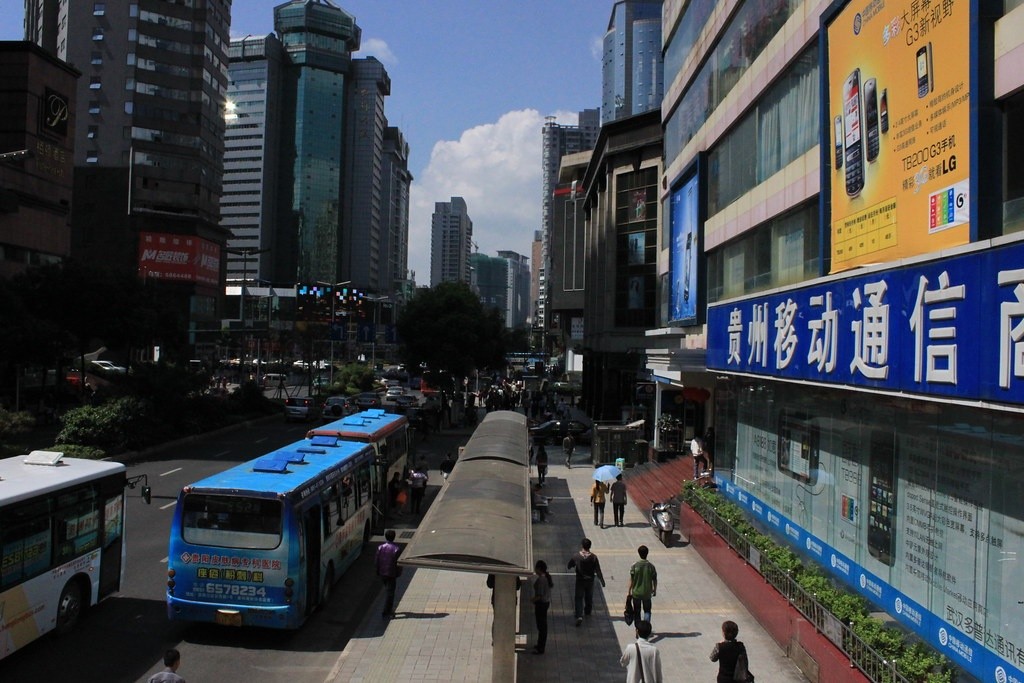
left=683, top=233, right=693, bottom=301
left=916, top=42, right=933, bottom=99
left=833, top=67, right=889, bottom=200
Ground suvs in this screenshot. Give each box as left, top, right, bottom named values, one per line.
left=320, top=396, right=359, bottom=424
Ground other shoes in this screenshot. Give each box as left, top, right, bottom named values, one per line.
left=534, top=645, right=537, bottom=649
left=576, top=617, right=582, bottom=626
left=585, top=613, right=591, bottom=618
left=533, top=650, right=544, bottom=655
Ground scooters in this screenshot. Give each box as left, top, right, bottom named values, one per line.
left=649, top=494, right=676, bottom=548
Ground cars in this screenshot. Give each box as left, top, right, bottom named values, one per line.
left=313, top=378, right=329, bottom=389
left=385, top=380, right=419, bottom=411
left=526, top=364, right=549, bottom=371
left=355, top=393, right=382, bottom=409
left=530, top=420, right=592, bottom=447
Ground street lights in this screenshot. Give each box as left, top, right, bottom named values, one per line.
left=361, top=296, right=388, bottom=371
left=317, top=281, right=351, bottom=386
left=220, top=249, right=270, bottom=364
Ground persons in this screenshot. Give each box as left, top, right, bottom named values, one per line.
left=691, top=427, right=715, bottom=480
left=529, top=441, right=555, bottom=523
left=563, top=433, right=575, bottom=469
left=567, top=538, right=605, bottom=626
left=709, top=621, right=748, bottom=683
left=590, top=474, right=626, bottom=528
left=480, top=384, right=565, bottom=419
left=628, top=546, right=658, bottom=637
left=211, top=375, right=226, bottom=389
left=366, top=451, right=456, bottom=616
left=146, top=649, right=186, bottom=683
left=620, top=621, right=662, bottom=683
left=533, top=560, right=554, bottom=652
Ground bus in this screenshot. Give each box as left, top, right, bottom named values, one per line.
left=0, top=451, right=151, bottom=660
left=305, top=409, right=417, bottom=516
left=506, top=353, right=551, bottom=365
left=166, top=437, right=386, bottom=632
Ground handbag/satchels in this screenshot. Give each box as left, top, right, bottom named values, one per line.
left=624, top=595, right=635, bottom=626
left=734, top=642, right=751, bottom=683
left=396, top=492, right=407, bottom=503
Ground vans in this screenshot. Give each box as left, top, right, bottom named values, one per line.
left=263, top=374, right=287, bottom=387
left=285, top=397, right=316, bottom=422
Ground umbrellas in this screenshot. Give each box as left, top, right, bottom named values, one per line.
left=592, top=465, right=622, bottom=482
